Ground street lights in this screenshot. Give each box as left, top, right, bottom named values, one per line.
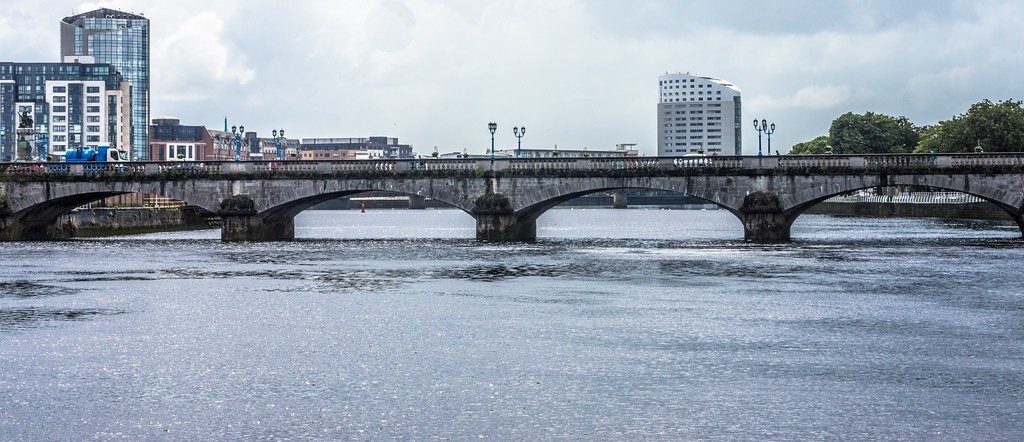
left=753, top=118, right=766, bottom=155
left=488, top=122, right=497, bottom=158
left=513, top=126, right=525, bottom=158
left=763, top=122, right=775, bottom=155
left=232, top=125, right=244, bottom=161
left=272, top=129, right=284, bottom=160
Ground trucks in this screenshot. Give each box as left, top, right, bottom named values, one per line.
left=66, top=143, right=130, bottom=167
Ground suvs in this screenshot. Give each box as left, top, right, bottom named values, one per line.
left=674, top=154, right=712, bottom=167
